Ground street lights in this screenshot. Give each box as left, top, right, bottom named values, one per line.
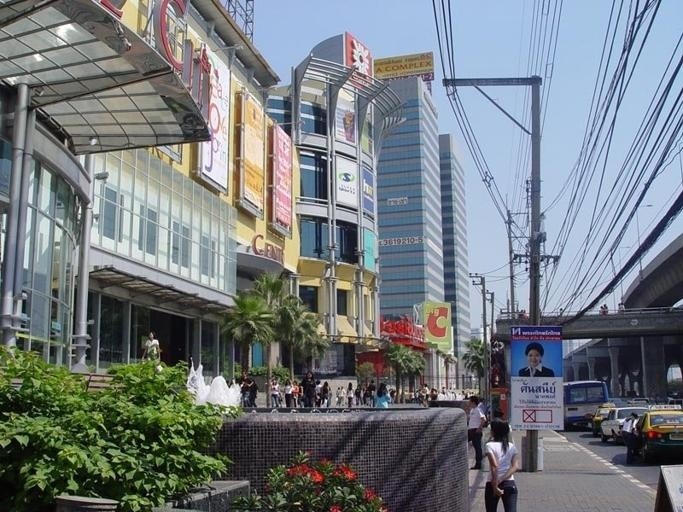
left=616, top=245, right=632, bottom=297
left=0, top=77, right=44, bottom=356
left=635, top=203, right=654, bottom=272
left=68, top=169, right=111, bottom=376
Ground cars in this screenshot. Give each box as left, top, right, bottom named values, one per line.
left=631, top=404, right=682, bottom=461
left=590, top=396, right=682, bottom=414
left=590, top=403, right=615, bottom=437
left=598, top=405, right=648, bottom=444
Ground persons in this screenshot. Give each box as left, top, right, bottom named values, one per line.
left=618, top=303, right=621, bottom=311
left=518, top=310, right=525, bottom=319
left=621, top=413, right=638, bottom=465
left=141, top=330, right=162, bottom=367
left=465, top=395, right=484, bottom=471
left=559, top=308, right=562, bottom=316
left=603, top=303, right=608, bottom=314
left=483, top=417, right=522, bottom=512
left=599, top=306, right=604, bottom=315
left=518, top=343, right=554, bottom=376
left=620, top=303, right=624, bottom=311
left=234, top=367, right=474, bottom=409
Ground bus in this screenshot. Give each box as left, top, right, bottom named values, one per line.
left=563, top=380, right=608, bottom=428
